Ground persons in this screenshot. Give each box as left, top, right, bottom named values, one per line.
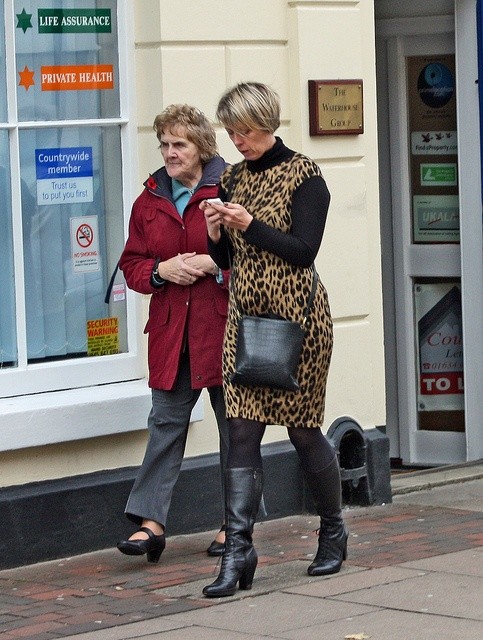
left=117, top=103, right=233, bottom=563
left=197, top=81, right=348, bottom=598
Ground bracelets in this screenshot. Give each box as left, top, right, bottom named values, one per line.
left=152, top=256, right=164, bottom=283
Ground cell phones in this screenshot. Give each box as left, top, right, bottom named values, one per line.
left=207, top=197, right=225, bottom=206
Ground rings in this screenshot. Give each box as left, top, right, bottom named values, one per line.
left=187, top=274, right=192, bottom=282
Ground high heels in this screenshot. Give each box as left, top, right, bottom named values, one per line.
left=307, top=456, right=349, bottom=576
left=207, top=525, right=227, bottom=555
left=203, top=466, right=263, bottom=597
left=117, top=525, right=166, bottom=563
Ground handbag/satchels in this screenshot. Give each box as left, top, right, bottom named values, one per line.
left=230, top=316, right=302, bottom=390
